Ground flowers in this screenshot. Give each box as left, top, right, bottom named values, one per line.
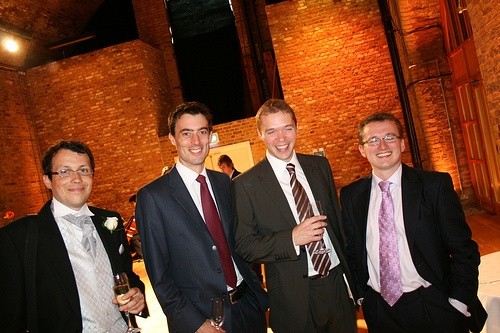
left=104, top=216, right=119, bottom=233
left=4, top=211, right=15, bottom=223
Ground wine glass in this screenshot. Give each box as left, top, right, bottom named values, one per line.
left=111, top=272, right=141, bottom=333
left=210, top=298, right=225, bottom=331
left=308, top=201, right=331, bottom=254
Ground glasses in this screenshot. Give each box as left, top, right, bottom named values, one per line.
left=50, top=167, right=93, bottom=177
left=362, top=134, right=402, bottom=147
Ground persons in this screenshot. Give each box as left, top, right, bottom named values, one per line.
left=134, top=101, right=270, bottom=333
left=217, top=155, right=242, bottom=179
left=0, top=139, right=149, bottom=333
left=128, top=193, right=143, bottom=262
left=339, top=111, right=489, bottom=333
left=232, top=99, right=360, bottom=333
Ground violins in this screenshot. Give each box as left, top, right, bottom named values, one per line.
left=125, top=215, right=137, bottom=244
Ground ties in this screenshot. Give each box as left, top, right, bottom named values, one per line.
left=378, top=180, right=402, bottom=307
left=76, top=213, right=98, bottom=258
left=286, top=161, right=332, bottom=277
left=195, top=174, right=237, bottom=290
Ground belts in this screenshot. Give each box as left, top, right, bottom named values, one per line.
left=226, top=279, right=247, bottom=304
